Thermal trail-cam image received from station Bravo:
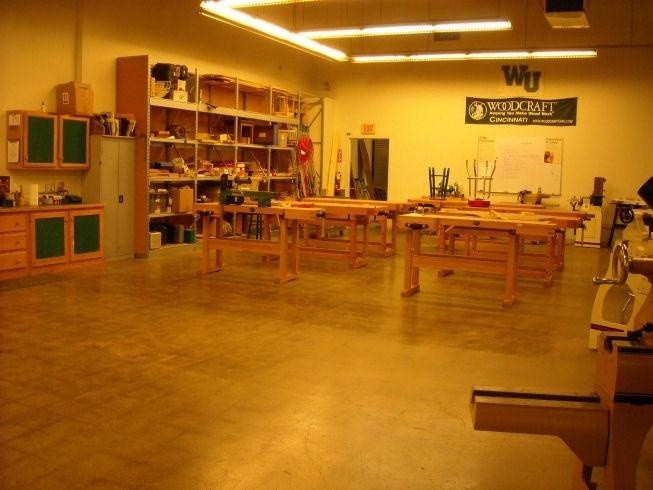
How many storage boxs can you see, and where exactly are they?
[172,187,194,214]
[169,89,188,103]
[149,232,162,250]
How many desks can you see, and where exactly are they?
[184,195,441,282]
[398,198,595,306]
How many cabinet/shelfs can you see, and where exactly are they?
[4,109,91,172]
[113,53,304,261]
[0,209,31,281]
[32,204,106,275]
[81,136,139,264]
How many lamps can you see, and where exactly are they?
[196,0,598,67]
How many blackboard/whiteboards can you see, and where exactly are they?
[475,135,564,196]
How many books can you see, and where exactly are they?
[150,173,178,178]
[150,220,196,245]
[92,109,136,137]
[148,168,169,173]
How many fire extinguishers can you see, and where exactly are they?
[335,172,341,194]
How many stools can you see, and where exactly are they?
[428,164,449,201]
[464,158,497,209]
